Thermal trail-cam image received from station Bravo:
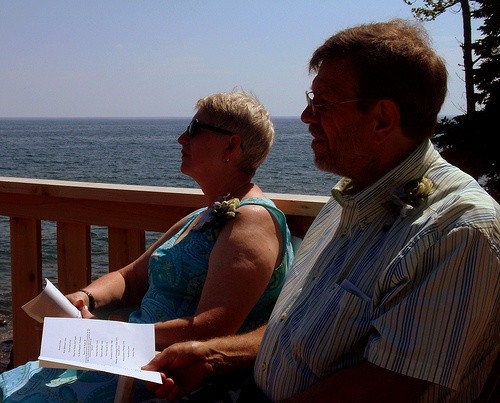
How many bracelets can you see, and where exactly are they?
[79,289,95,313]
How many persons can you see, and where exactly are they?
[0,87,295,403]
[140,19,500,403]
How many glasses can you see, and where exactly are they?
[187,118,245,152]
[305,91,360,116]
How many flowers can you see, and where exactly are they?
[377,176,434,234]
[198,194,241,234]
